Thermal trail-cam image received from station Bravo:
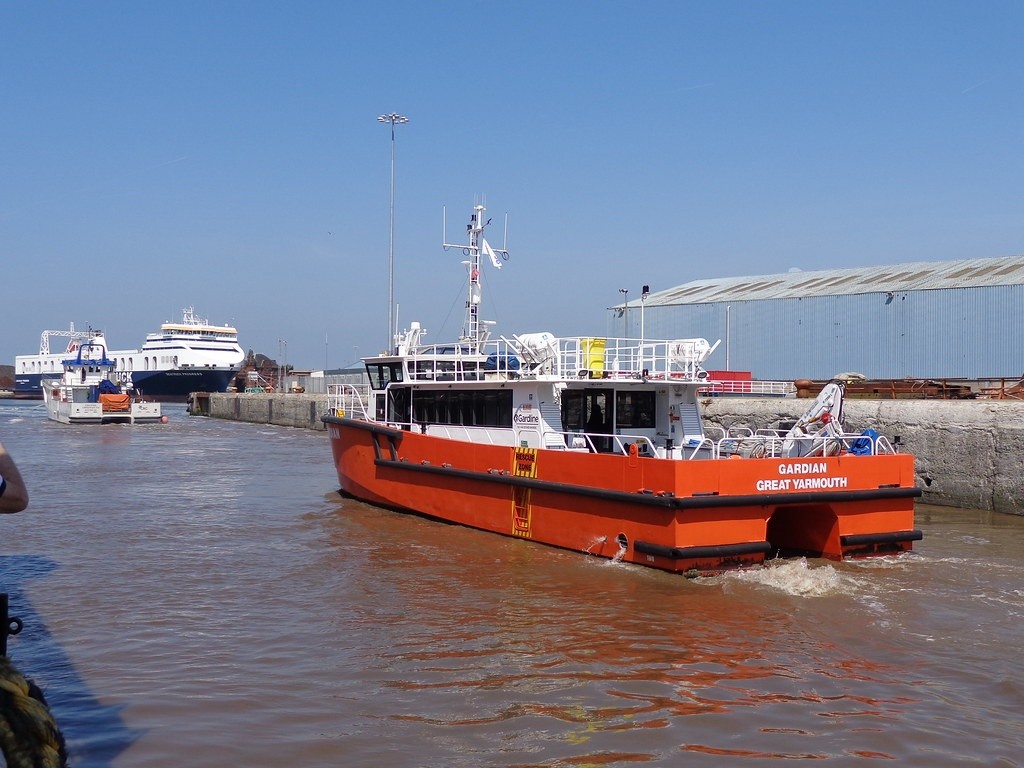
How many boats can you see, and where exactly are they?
[14,305,247,402]
[40,342,162,424]
[321,195,933,576]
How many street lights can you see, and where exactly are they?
[377,112,410,354]
[619,288,628,375]
[277,339,287,393]
